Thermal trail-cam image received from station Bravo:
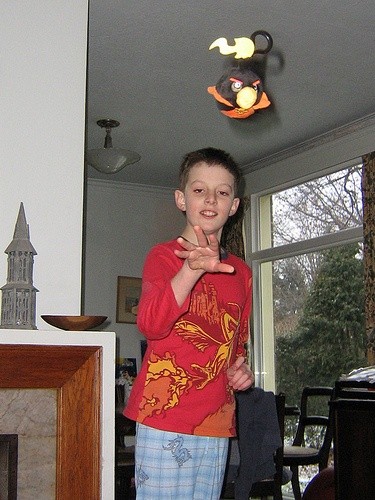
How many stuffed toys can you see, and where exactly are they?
[208,57,271,119]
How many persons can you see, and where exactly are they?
[121,146,255,500]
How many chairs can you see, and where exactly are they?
[253,394,288,500]
[275,385,336,500]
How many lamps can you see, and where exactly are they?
[206,30,274,119]
[85,118,141,175]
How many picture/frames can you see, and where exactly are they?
[115,356,137,407]
[116,275,143,325]
